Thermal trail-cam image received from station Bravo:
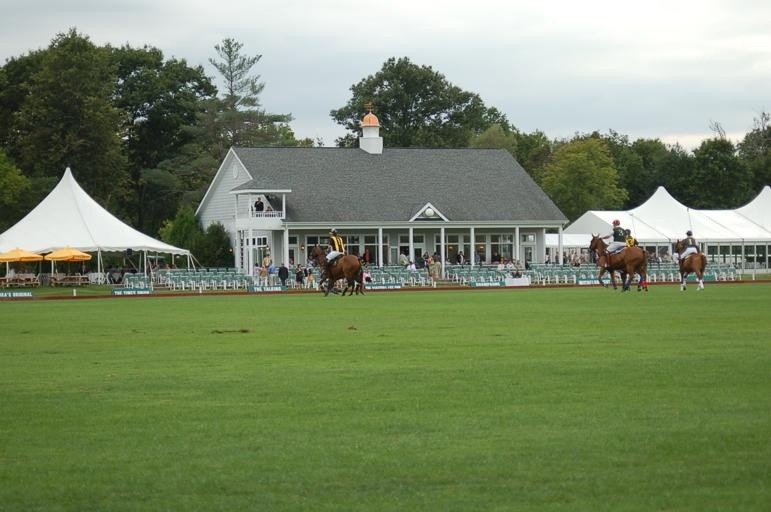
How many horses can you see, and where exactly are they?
[588,233,649,291]
[309,243,366,296]
[674,239,707,292]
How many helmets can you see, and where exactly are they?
[329,229,337,234]
[613,220,619,225]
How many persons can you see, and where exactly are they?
[323,227,344,265]
[396,249,530,277]
[253,197,263,217]
[262,206,275,217]
[363,248,371,266]
[623,229,640,247]
[248,205,253,216]
[102,261,176,281]
[600,219,628,266]
[545,249,675,268]
[251,250,317,288]
[678,230,698,260]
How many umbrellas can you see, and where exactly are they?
[0,248,43,282]
[43,246,92,276]
